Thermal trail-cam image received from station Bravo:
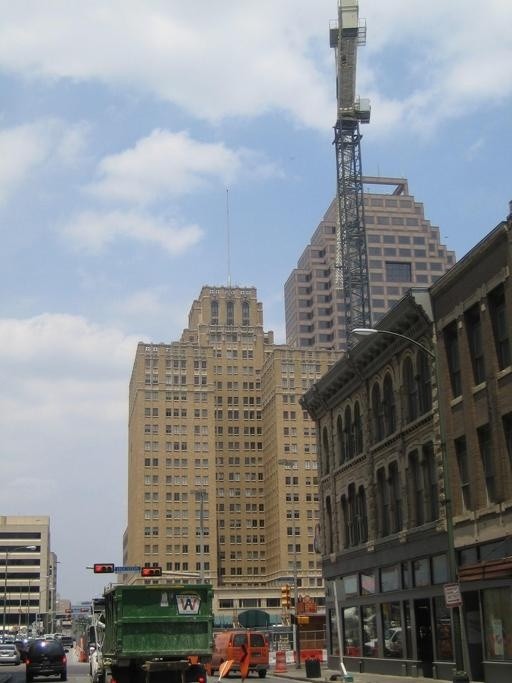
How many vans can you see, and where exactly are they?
[209,629,270,677]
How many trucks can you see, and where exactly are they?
[88,581,216,682]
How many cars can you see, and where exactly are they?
[0,627,101,681]
[365,623,412,658]
[437,621,482,660]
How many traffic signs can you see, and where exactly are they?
[114,567,140,574]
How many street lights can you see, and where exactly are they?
[351,325,434,359]
[2,543,37,644]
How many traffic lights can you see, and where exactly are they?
[140,566,162,576]
[280,583,291,608]
[93,563,115,573]
